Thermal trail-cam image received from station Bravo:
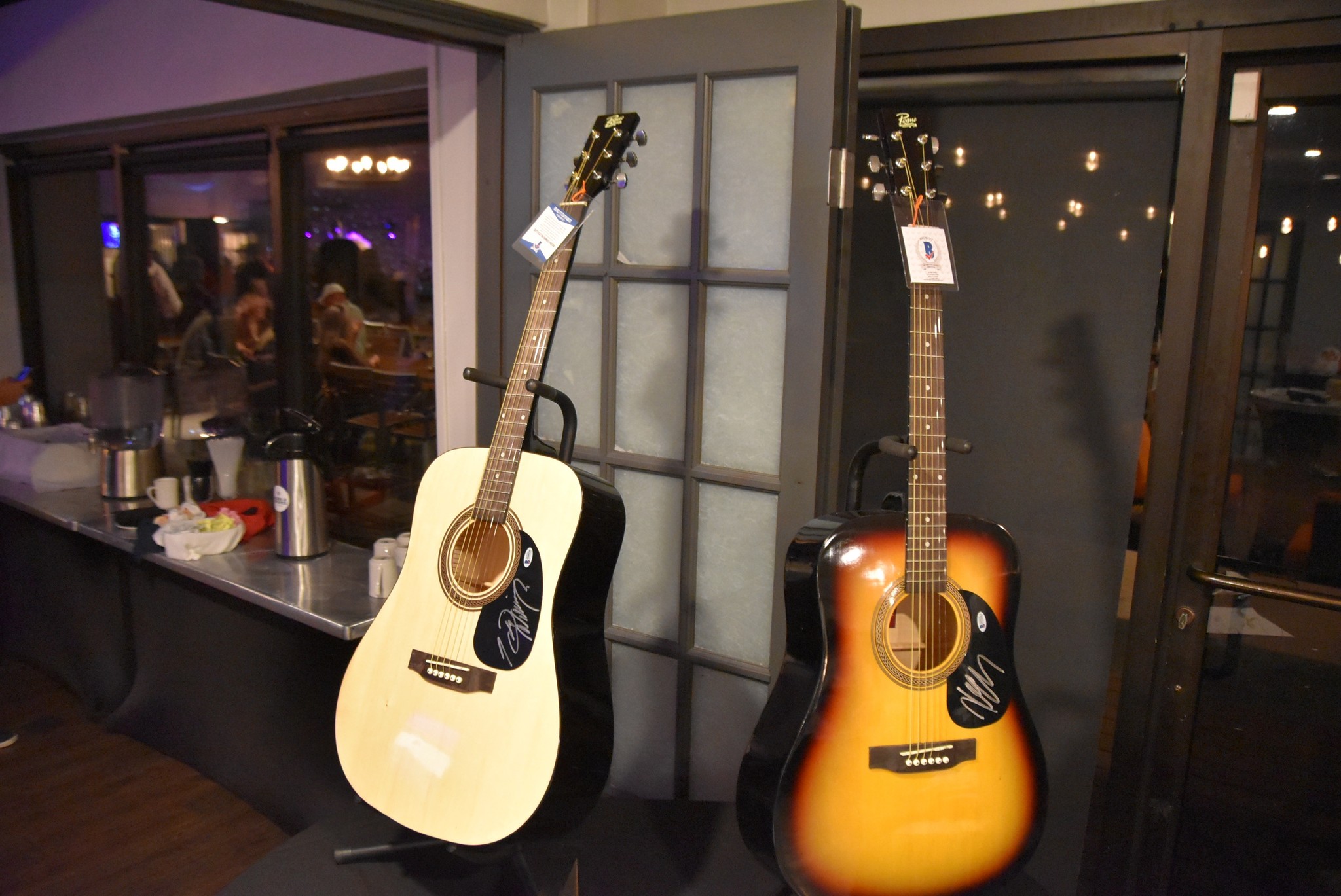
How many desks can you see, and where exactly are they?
[0,485,392,835]
[366,353,436,391]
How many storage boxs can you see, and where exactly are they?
[89,378,159,426]
[0,422,105,491]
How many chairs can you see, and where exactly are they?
[248,321,436,470]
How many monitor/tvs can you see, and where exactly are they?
[100,222,122,249]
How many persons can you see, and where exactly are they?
[113,217,382,368]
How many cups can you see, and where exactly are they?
[205,434,245,503]
[181,475,214,504]
[367,533,410,600]
[146,477,179,510]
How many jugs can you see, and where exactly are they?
[264,432,329,561]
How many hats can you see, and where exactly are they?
[317,283,345,303]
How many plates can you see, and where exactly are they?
[115,522,137,531]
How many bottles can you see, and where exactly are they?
[0,395,47,429]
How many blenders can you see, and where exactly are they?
[86,362,165,499]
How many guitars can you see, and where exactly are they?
[733,103,1052,896]
[331,113,648,847]
